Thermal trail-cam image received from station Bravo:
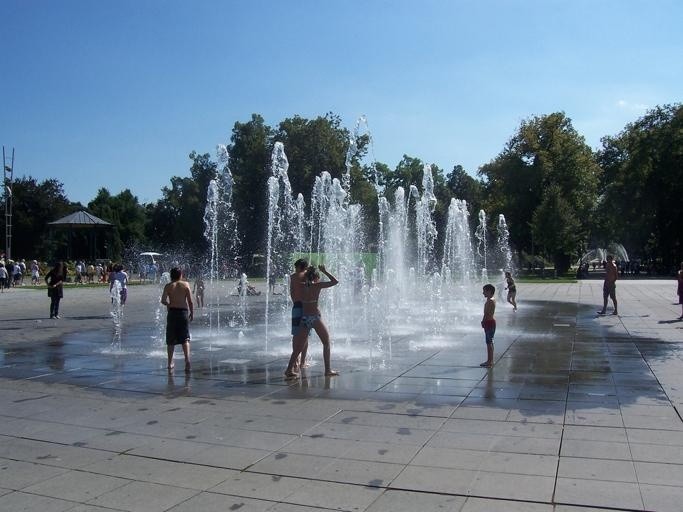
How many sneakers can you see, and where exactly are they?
[50,316,57,319]
[55,315,60,319]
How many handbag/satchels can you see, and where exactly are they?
[15,268,21,279]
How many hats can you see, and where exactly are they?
[10,260,14,263]
[15,262,18,265]
[21,259,25,262]
[34,260,37,263]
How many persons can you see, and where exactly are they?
[128,260,241,284]
[160,267,195,369]
[290,259,309,373]
[597,254,618,315]
[44,262,63,319]
[114,265,128,305]
[576,259,601,280]
[677,262,683,304]
[1,258,27,289]
[504,271,517,311]
[63,260,112,284]
[107,264,119,304]
[480,284,497,367]
[619,260,641,277]
[192,274,205,308]
[30,260,40,284]
[285,263,339,377]
[269,270,276,293]
[237,271,261,296]
